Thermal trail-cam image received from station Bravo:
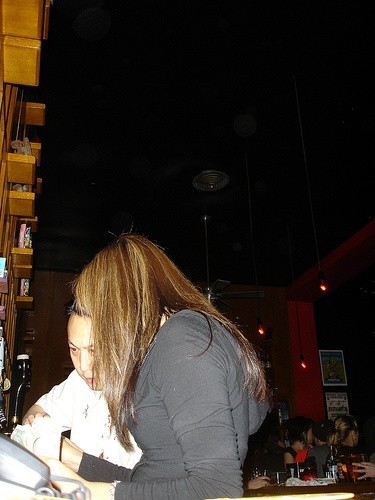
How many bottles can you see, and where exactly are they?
[8,354,32,432]
[326,445,339,484]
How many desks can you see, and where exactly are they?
[244,483,375,500]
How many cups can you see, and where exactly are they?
[276,472,287,484]
[262,470,270,477]
[345,451,370,483]
[303,464,317,481]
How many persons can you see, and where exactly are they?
[23,309,143,471]
[10,237,272,500]
[244,414,375,500]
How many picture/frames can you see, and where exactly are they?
[323,388,351,422]
[318,350,347,386]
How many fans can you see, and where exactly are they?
[193,217,266,311]
